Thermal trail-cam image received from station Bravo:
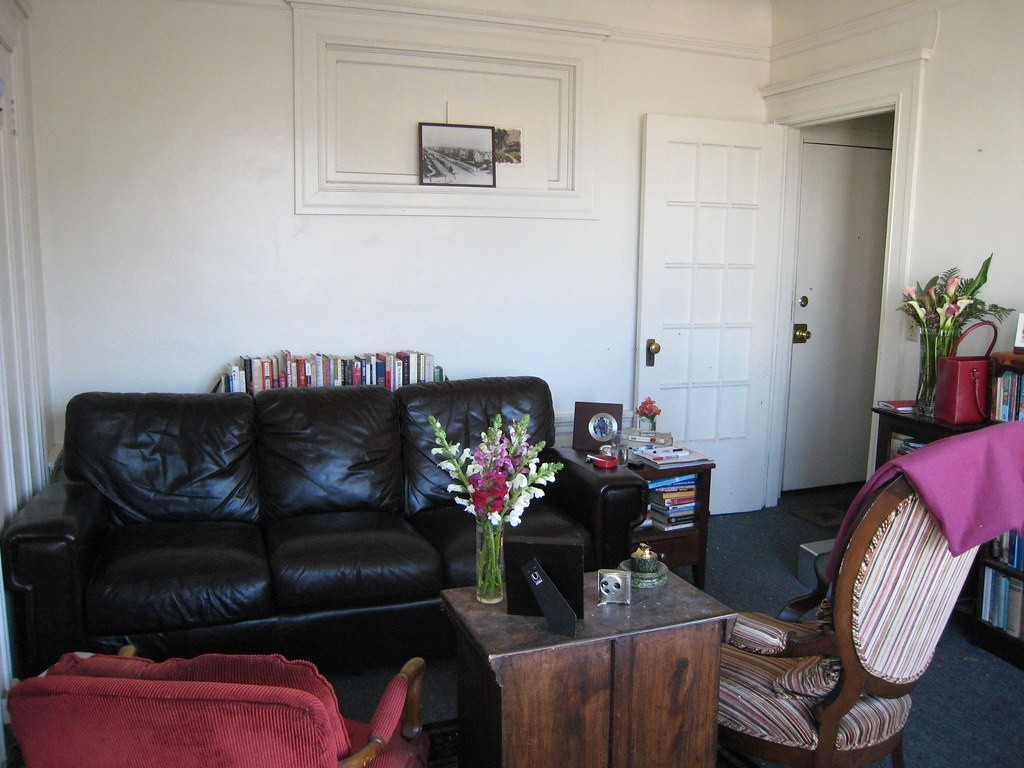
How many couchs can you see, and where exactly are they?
[3,377,591,673]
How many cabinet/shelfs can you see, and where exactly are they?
[868,351,1023,671]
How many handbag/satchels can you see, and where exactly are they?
[934,322,998,426]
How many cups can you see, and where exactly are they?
[611,431,629,466]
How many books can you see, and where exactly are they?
[625,427,715,533]
[219,350,446,391]
[995,372,1024,423]
[982,529,1023,638]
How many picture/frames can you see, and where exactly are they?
[504,536,584,619]
[419,122,496,188]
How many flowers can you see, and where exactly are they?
[895,252,1017,410]
[428,413,565,598]
[636,397,662,431]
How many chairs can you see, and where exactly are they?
[7,647,431,768]
[713,422,1024,768]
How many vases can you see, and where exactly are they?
[475,518,504,603]
[648,420,657,431]
[914,328,960,417]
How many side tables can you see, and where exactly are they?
[548,438,715,589]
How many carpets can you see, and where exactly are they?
[792,500,852,535]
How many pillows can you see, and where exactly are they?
[45,648,356,760]
[772,599,865,702]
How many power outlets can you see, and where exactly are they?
[905,317,921,341]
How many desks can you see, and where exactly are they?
[441,558,736,768]
